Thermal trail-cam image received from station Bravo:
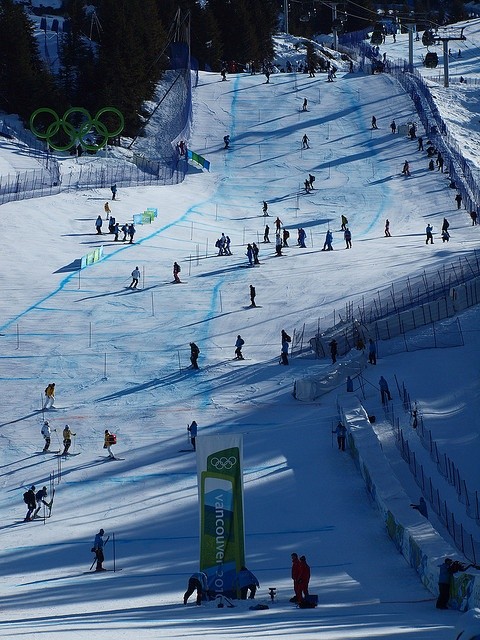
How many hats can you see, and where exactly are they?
[100,529,104,533]
[300,556,306,560]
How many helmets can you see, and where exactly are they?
[44,421,49,424]
[65,424,69,429]
[43,486,47,490]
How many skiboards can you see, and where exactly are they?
[226,357,252,361]
[99,456,125,461]
[124,242,139,244]
[33,407,65,412]
[239,265,260,268]
[167,282,188,284]
[269,255,287,257]
[54,453,80,456]
[32,516,50,518]
[35,450,60,453]
[179,449,195,451]
[84,568,122,574]
[15,519,46,522]
[243,305,262,309]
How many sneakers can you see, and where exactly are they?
[50,406,55,408]
[43,407,48,409]
[96,567,103,571]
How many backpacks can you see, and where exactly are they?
[299,599,317,608]
[109,434,117,444]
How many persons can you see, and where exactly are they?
[435,558,454,610]
[187,420,198,449]
[189,342,200,369]
[332,420,347,451]
[178,140,186,155]
[234,335,244,359]
[273,217,283,233]
[109,216,115,234]
[427,140,434,145]
[449,179,458,189]
[328,337,338,363]
[173,262,182,283]
[371,116,378,129]
[279,337,289,365]
[223,135,232,149]
[390,121,396,133]
[282,228,289,247]
[237,565,260,600]
[281,329,287,345]
[300,230,306,248]
[104,202,111,220]
[297,228,301,245]
[410,126,416,141]
[436,157,445,173]
[111,184,117,200]
[23,484,36,522]
[40,420,57,454]
[426,146,435,156]
[128,265,141,289]
[460,76,464,83]
[442,217,449,235]
[290,553,303,605]
[225,236,231,254]
[323,230,331,250]
[103,429,117,461]
[455,195,462,209]
[93,529,106,571]
[250,284,257,307]
[434,147,440,153]
[276,233,282,256]
[418,137,424,151]
[379,375,392,403]
[302,133,310,149]
[441,231,450,243]
[401,159,410,176]
[356,338,365,350]
[449,48,452,56]
[385,219,391,237]
[183,572,209,605]
[61,424,76,456]
[471,210,478,226]
[264,224,270,242]
[308,173,315,190]
[218,232,229,255]
[457,48,462,56]
[346,375,353,393]
[112,223,121,241]
[33,486,53,519]
[122,224,128,240]
[392,33,396,44]
[436,150,441,166]
[252,242,259,264]
[128,224,135,243]
[344,228,352,249]
[296,555,310,597]
[246,243,254,266]
[95,215,102,234]
[368,338,376,365]
[303,178,310,194]
[302,97,308,111]
[217,44,387,84]
[426,224,434,244]
[428,158,435,171]
[340,214,347,230]
[262,200,269,216]
[43,382,56,410]
